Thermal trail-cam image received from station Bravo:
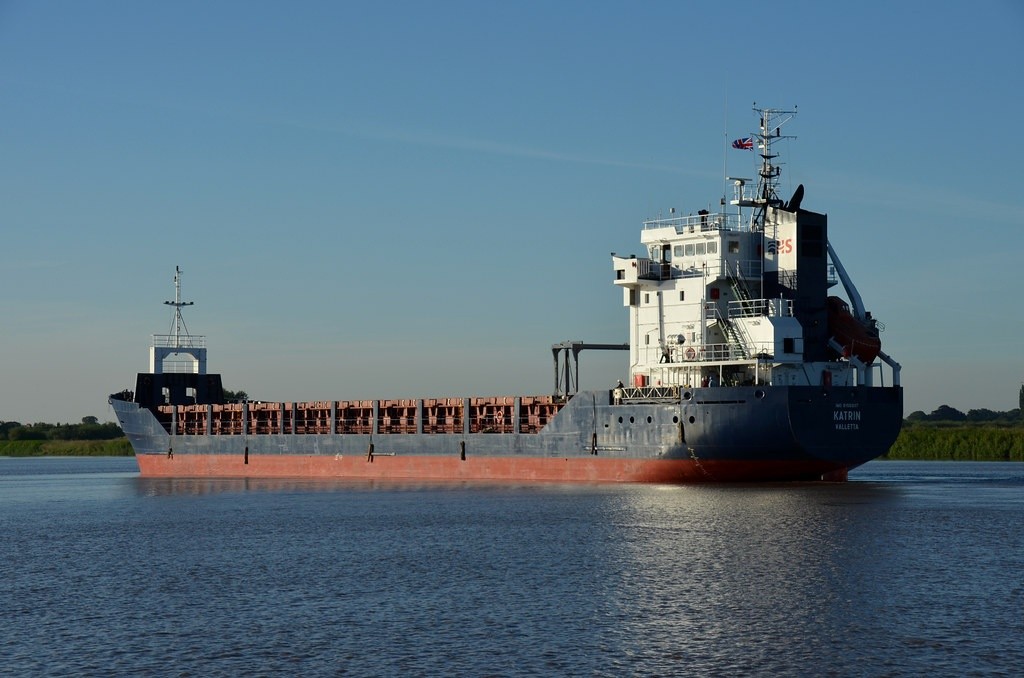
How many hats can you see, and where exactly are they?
[617,380,621,383]
[709,376,712,380]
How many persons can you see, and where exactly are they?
[616,380,624,388]
[702,376,719,388]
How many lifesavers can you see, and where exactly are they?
[685,348,695,359]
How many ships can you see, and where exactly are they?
[108,91,904,481]
